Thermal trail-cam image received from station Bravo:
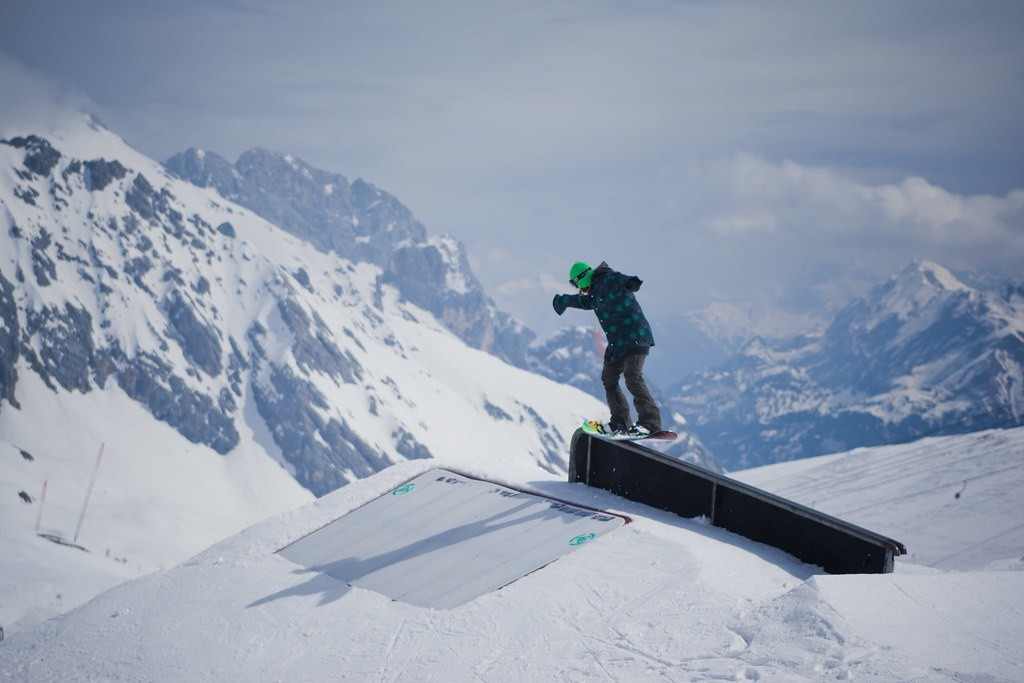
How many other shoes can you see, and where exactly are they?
[620,421,651,437]
[595,422,625,436]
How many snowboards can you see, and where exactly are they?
[581,419,678,441]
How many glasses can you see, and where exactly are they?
[569,279,578,288]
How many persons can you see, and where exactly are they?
[553,261,661,435]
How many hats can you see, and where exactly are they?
[569,261,594,289]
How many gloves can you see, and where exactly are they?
[626,276,643,292]
